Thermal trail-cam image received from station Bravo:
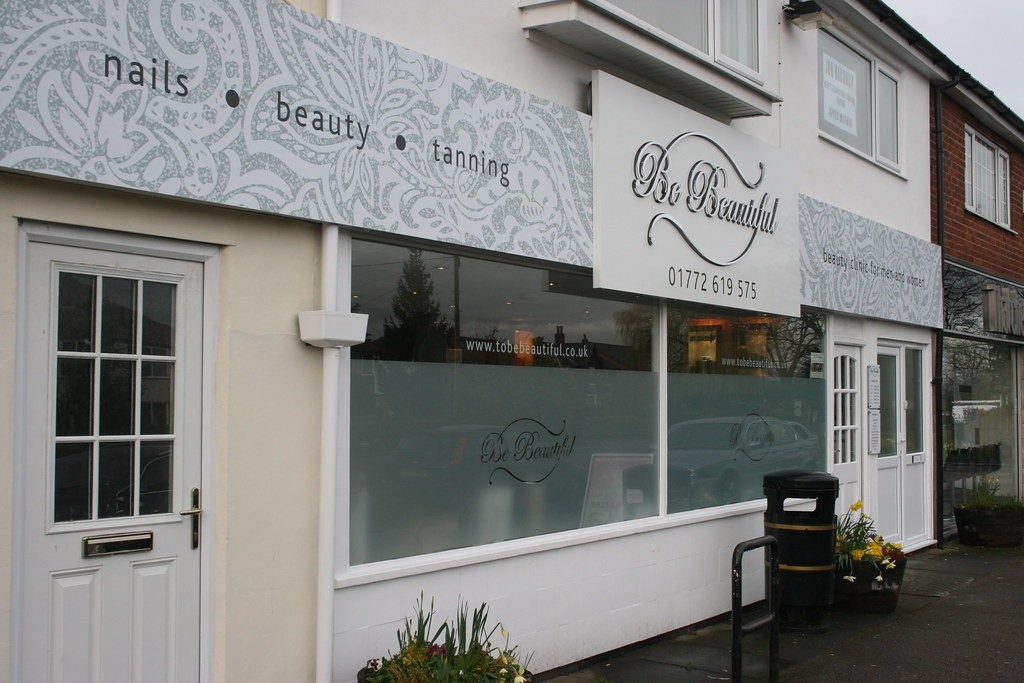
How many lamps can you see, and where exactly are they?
[515,328,535,367]
[783,0,834,31]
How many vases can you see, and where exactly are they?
[953,505,1024,548]
[834,558,907,615]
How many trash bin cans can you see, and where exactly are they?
[761,471,839,631]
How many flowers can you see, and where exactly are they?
[973,472,1001,507]
[835,500,908,583]
[356,590,540,683]
